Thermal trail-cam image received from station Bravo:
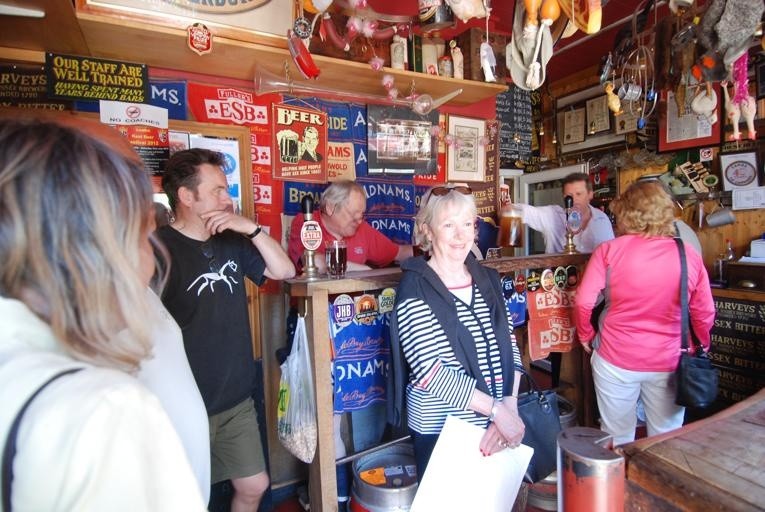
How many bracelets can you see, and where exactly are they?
[246,223,262,238]
[509,394,519,399]
[487,398,503,423]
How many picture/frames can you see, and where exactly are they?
[445,112,487,184]
[717,149,762,192]
[656,78,725,156]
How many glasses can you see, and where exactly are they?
[343,205,364,222]
[425,186,472,207]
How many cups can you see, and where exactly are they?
[495,204,524,249]
[705,202,735,228]
[325,240,347,279]
[279,136,301,164]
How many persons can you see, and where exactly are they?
[145,148,298,512]
[285,177,420,512]
[625,177,704,426]
[495,169,617,392]
[298,125,324,163]
[572,177,717,449]
[51,115,216,512]
[394,181,534,512]
[0,109,213,512]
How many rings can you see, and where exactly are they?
[503,441,508,449]
[498,438,504,445]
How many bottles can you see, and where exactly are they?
[390,34,405,70]
[694,201,705,230]
[420,31,453,78]
[712,239,737,285]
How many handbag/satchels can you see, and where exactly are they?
[513,363,562,485]
[675,347,719,410]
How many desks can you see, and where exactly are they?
[281,246,593,511]
